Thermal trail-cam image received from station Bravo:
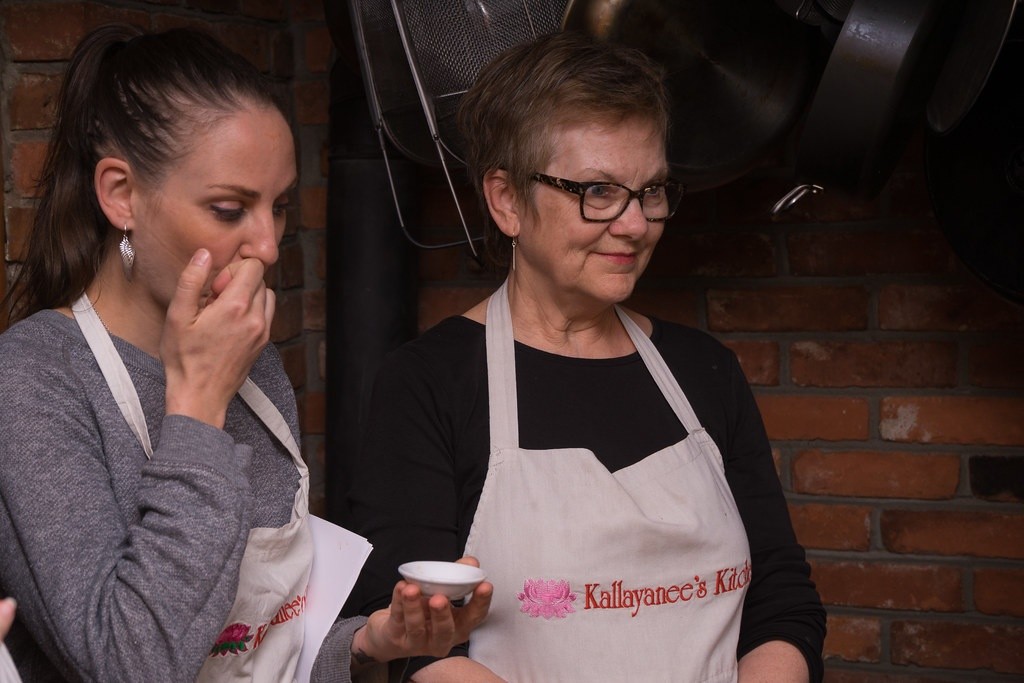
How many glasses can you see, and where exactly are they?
[529,168,685,221]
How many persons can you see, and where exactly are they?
[312,33,827,683]
[0,24,493,683]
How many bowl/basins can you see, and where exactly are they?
[398,560,487,601]
